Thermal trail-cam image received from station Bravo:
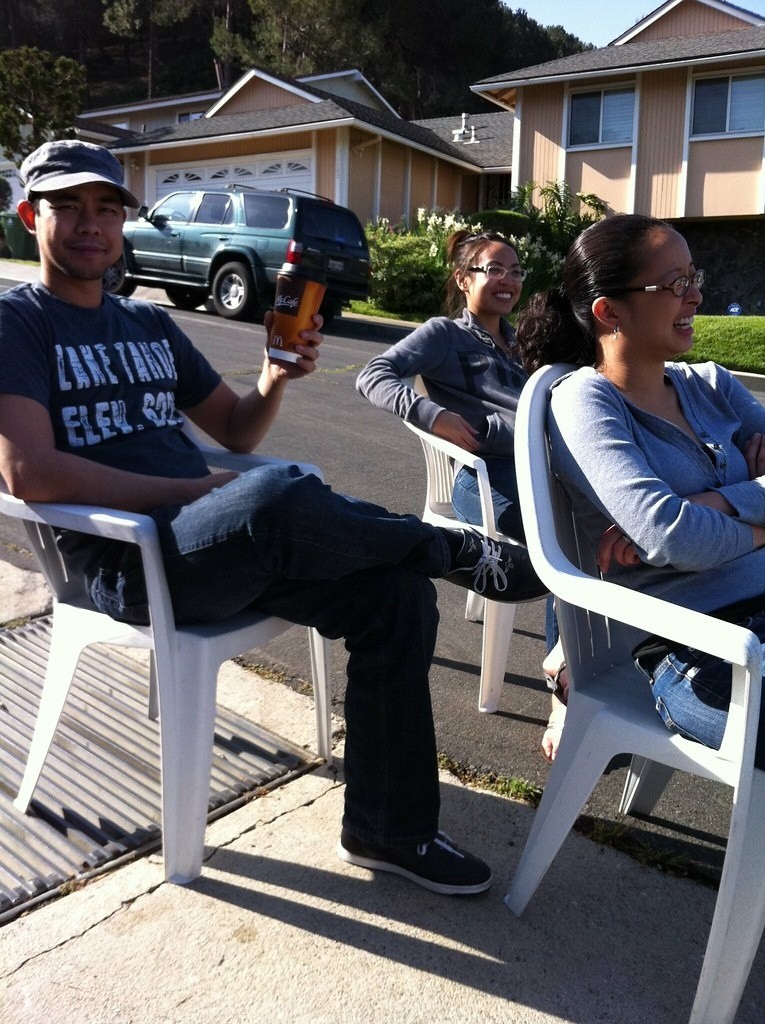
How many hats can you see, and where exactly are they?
[19,139,139,211]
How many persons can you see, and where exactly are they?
[549,213,763,770]
[1,139,553,895]
[356,230,606,765]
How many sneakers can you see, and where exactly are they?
[340,827,493,895]
[441,526,554,605]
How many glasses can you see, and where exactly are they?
[461,266,527,281]
[621,268,705,296]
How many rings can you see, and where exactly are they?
[622,533,632,544]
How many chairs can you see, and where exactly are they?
[211,204,226,221]
[0,406,333,885]
[505,359,765,1023]
[398,367,526,714]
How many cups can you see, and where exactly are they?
[267,259,330,365]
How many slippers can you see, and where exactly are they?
[541,661,569,705]
[539,720,566,764]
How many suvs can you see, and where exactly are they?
[102,185,378,326]
